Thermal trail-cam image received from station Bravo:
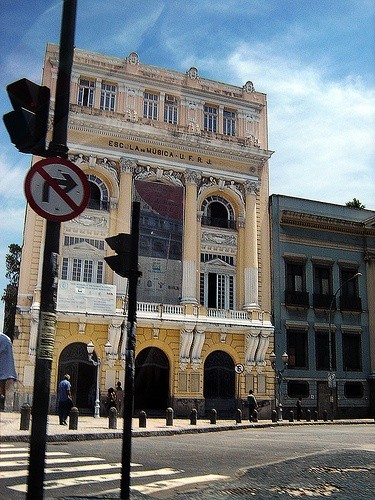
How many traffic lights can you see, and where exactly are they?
[103,233,131,277]
[2,77,51,155]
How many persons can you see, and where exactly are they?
[247,390,258,421]
[58,374,73,426]
[295,400,302,422]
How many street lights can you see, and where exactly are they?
[328,273,362,420]
[86,340,112,418]
[269,351,288,420]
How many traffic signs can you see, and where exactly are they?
[234,363,244,374]
